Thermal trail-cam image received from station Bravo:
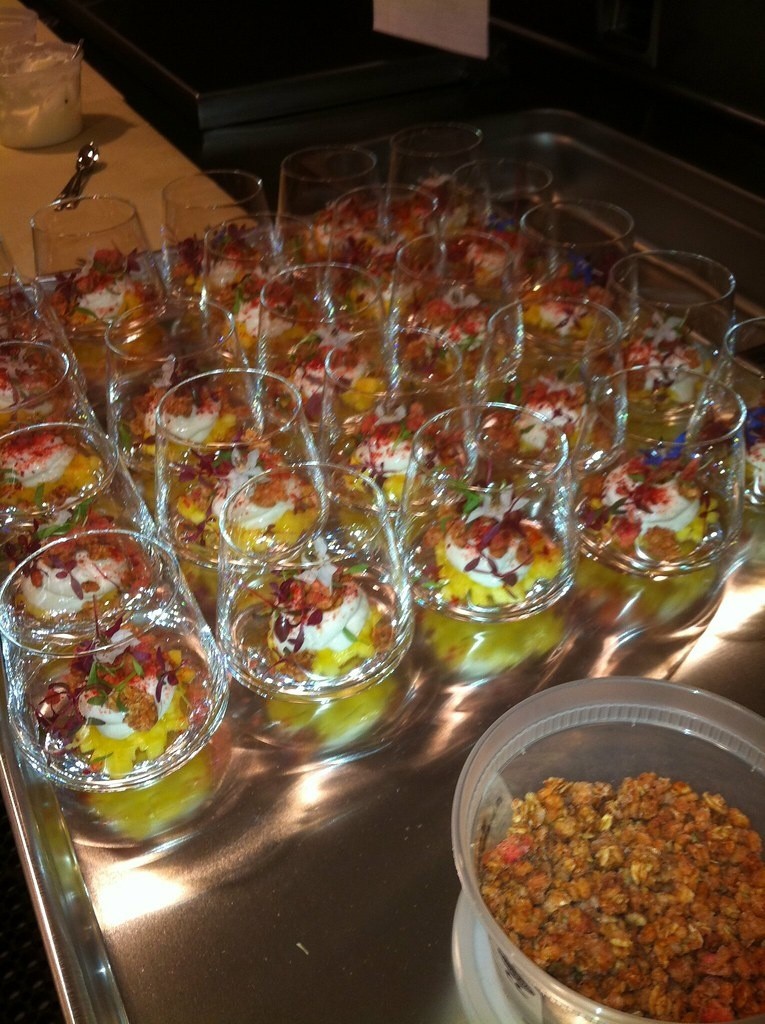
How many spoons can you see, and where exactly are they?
[65,144,99,208]
[50,143,94,211]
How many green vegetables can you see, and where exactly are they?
[0,197,699,763]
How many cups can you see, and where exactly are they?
[0,4,38,54]
[1,117,764,850]
[0,39,82,149]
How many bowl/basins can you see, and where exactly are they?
[452,675,765,1023]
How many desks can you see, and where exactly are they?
[1,0,257,279]
